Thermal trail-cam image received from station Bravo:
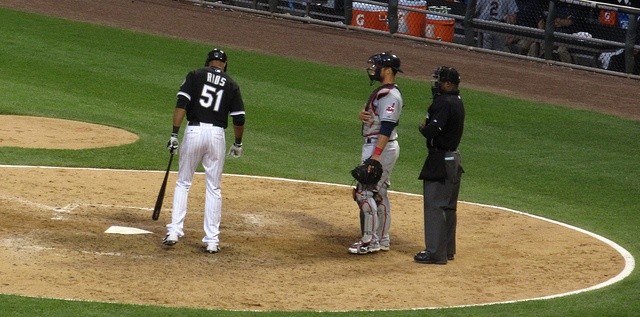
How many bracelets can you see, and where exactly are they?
[173,126,179,133]
[373,146,383,155]
[235,137,241,144]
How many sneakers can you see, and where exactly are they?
[379,238,389,251]
[348,241,380,253]
[205,243,218,253]
[162,234,178,246]
[416,250,453,260]
[414,254,448,264]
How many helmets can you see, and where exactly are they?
[205,48,227,72]
[366,51,400,80]
[430,66,461,95]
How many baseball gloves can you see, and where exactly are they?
[350,159,383,185]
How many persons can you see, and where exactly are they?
[162,48,245,253]
[527,2,576,63]
[348,50,404,255]
[473,0,519,52]
[414,65,465,265]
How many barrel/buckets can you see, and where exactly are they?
[397,0,425,37]
[350,2,387,30]
[424,14,454,42]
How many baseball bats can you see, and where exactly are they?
[153,154,173,220]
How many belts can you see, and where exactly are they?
[188,121,216,126]
[364,133,398,143]
[428,147,458,152]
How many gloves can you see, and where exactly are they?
[166,133,178,156]
[228,142,243,158]
